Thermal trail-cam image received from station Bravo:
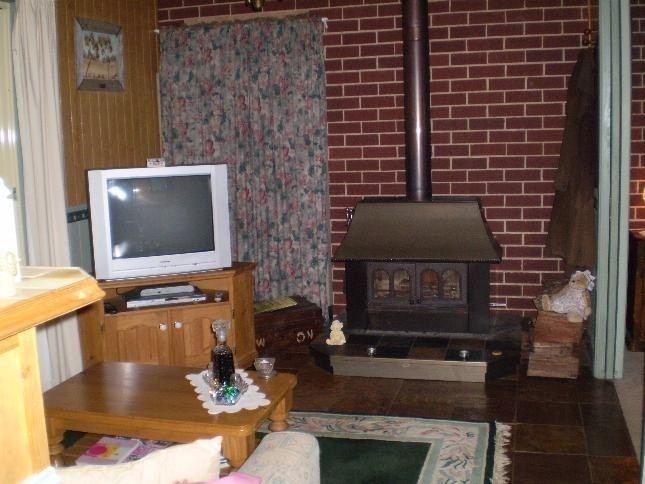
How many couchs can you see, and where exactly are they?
[22,431,321,483]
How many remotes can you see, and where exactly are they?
[104,302,116,313]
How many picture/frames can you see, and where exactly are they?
[73,16,125,92]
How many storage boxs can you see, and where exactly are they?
[521,305,589,380]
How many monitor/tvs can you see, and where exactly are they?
[87,162,233,281]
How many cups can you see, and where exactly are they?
[254,356,275,379]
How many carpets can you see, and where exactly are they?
[62,410,514,484]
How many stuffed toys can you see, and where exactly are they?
[542,269,595,322]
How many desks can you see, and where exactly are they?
[626,229,645,352]
[0,264,106,483]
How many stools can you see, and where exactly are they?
[253,295,325,365]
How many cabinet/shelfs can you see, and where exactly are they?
[78,261,258,375]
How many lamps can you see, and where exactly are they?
[247,0,276,10]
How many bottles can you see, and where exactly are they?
[210,319,235,387]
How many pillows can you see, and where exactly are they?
[61,436,224,483]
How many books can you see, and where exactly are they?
[76,436,164,467]
